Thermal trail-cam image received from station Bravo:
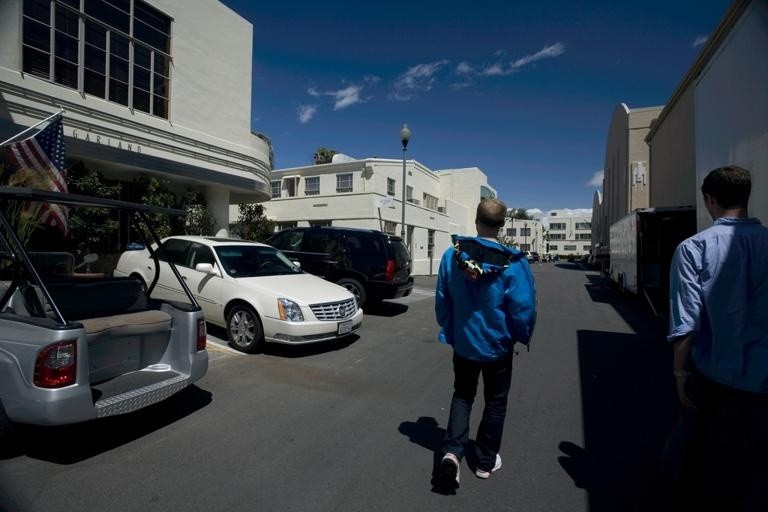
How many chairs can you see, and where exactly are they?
[21,253,141,317]
[240,249,271,272]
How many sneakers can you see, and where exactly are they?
[475,454,502,479]
[440,453,460,489]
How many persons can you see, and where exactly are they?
[434,197,539,491]
[665,164,768,512]
[543,252,560,266]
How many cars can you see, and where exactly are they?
[0,195,210,426]
[113,235,364,354]
[523,251,560,264]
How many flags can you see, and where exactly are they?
[6,115,69,238]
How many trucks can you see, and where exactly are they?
[609,207,696,317]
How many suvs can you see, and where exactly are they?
[255,225,412,307]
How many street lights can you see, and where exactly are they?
[400,123,411,243]
[524,222,527,251]
